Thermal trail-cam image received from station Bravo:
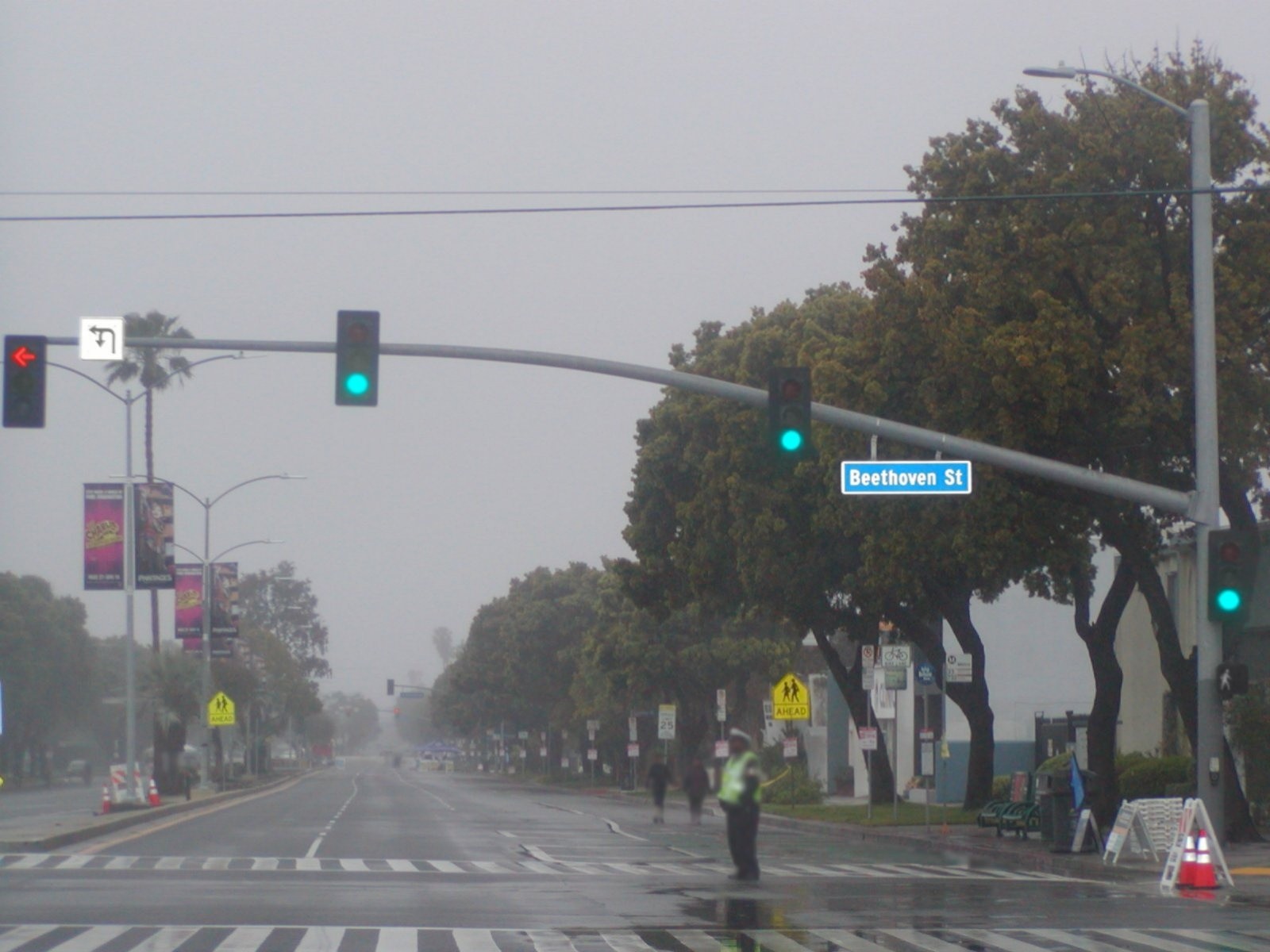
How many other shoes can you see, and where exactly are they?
[654,816,664,824]
[692,819,701,826]
[726,870,761,881]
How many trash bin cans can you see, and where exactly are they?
[1033,765,1099,854]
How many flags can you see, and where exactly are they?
[81,482,126,591]
[173,563,203,638]
[182,636,205,657]
[134,480,178,591]
[210,636,235,657]
[208,560,241,640]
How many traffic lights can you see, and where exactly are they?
[1208,529,1258,623]
[2,334,47,430]
[387,679,394,696]
[767,365,813,460]
[335,309,381,407]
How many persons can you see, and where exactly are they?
[646,753,679,826]
[717,726,763,880]
[682,756,712,827]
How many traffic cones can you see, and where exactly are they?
[1175,831,1197,884]
[1194,829,1222,889]
[147,778,164,807]
[100,785,113,815]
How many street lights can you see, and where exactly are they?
[1023,64,1228,825]
[51,350,317,800]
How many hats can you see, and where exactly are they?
[730,728,750,743]
